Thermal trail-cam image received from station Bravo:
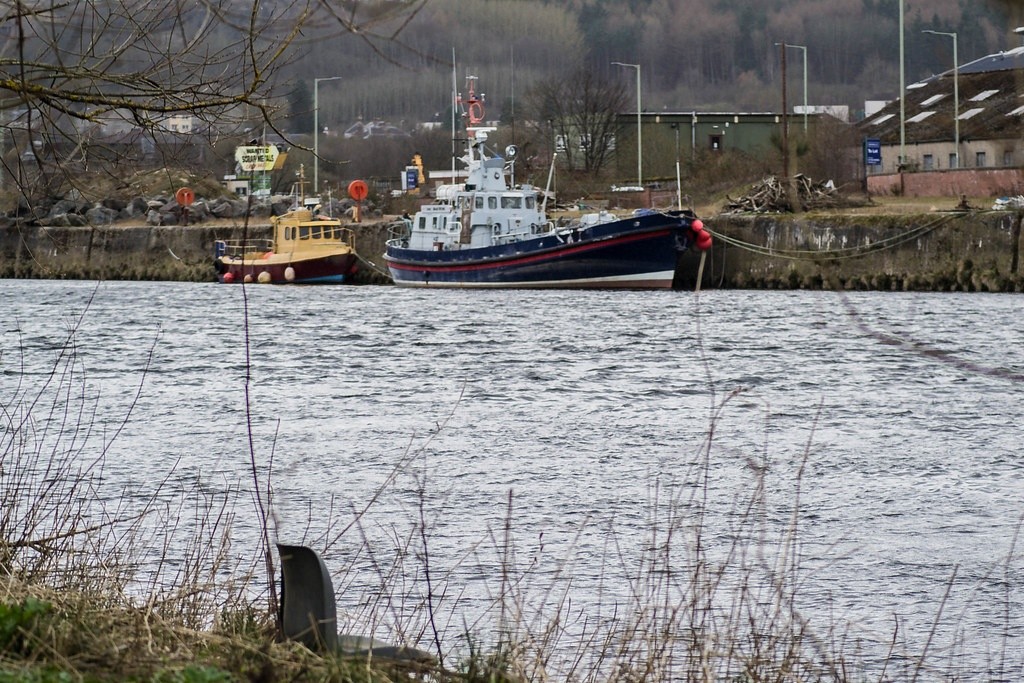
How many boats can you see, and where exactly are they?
[383,46,712,294]
[215,165,359,285]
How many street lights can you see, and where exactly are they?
[774,42,808,136]
[922,29,959,168]
[609,60,642,191]
[314,76,344,199]
